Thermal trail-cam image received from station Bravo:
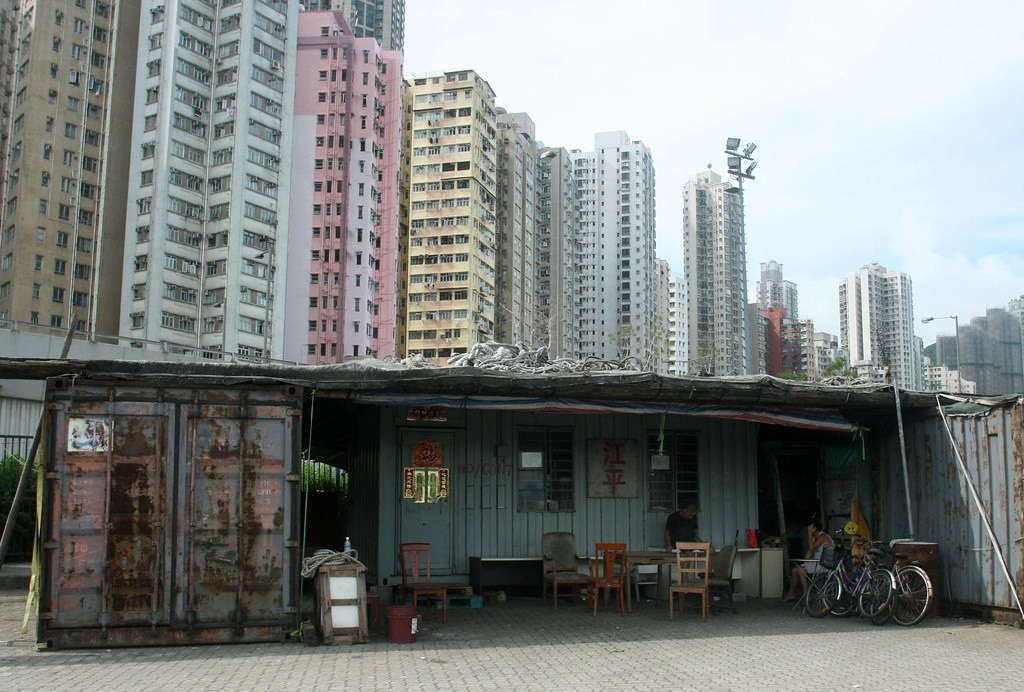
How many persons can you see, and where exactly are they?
[782,520,835,602]
[665,501,714,583]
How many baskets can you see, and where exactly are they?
[819,544,845,570]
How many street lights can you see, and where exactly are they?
[724,137,758,375]
[922,315,962,393]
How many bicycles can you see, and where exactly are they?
[804,526,933,626]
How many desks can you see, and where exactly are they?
[367,593,381,630]
[785,559,840,614]
[390,582,473,611]
[575,547,762,606]
[468,556,542,600]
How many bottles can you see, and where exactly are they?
[344,537,351,556]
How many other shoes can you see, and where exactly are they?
[780,595,797,602]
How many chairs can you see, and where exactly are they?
[669,542,739,620]
[586,543,629,618]
[543,532,594,611]
[397,543,446,624]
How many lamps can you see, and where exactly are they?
[403,406,447,422]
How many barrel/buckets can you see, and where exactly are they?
[894,541,939,620]
[386,606,418,643]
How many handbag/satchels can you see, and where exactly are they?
[746,528,758,547]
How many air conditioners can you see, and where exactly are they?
[25,10,32,16]
[44,173,50,177]
[56,11,63,17]
[18,66,23,72]
[45,145,52,150]
[10,172,17,177]
[89,2,283,318]
[14,144,19,150]
[52,63,59,71]
[47,117,54,123]
[51,90,58,95]
[54,37,61,45]
